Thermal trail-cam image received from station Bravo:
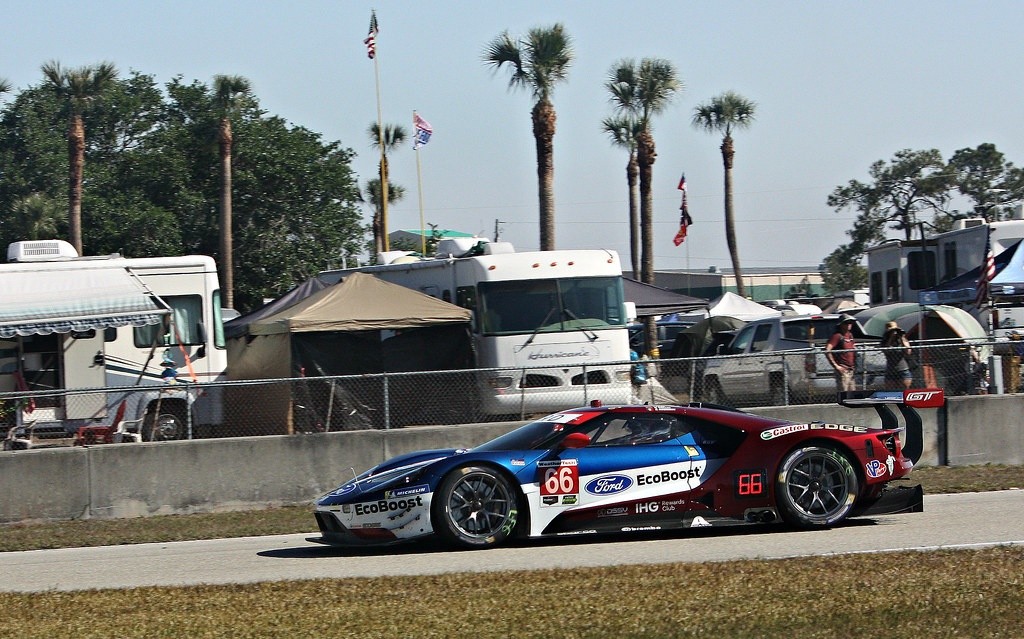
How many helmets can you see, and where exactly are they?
[621,416,672,446]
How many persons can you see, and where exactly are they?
[826,313,858,391]
[880,321,913,389]
[622,416,677,444]
[1005,331,1024,393]
[957,341,989,396]
[290,341,328,433]
[630,345,642,402]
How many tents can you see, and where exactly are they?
[223,274,483,437]
[621,275,782,393]
[918,238,1024,364]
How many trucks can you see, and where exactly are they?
[866,218,1023,355]
[0,239,228,443]
[316,235,637,418]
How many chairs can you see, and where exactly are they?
[112,394,149,443]
[78,400,127,444]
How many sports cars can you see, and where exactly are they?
[313,386,948,554]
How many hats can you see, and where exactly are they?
[836,314,857,326]
[885,322,902,332]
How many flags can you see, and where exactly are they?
[673,174,693,246]
[363,8,380,59]
[974,224,996,308]
[413,112,433,150]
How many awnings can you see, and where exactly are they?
[0,270,173,385]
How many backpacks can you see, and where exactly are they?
[631,365,647,385]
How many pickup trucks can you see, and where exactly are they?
[702,312,889,409]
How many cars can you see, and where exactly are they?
[626,319,698,359]
